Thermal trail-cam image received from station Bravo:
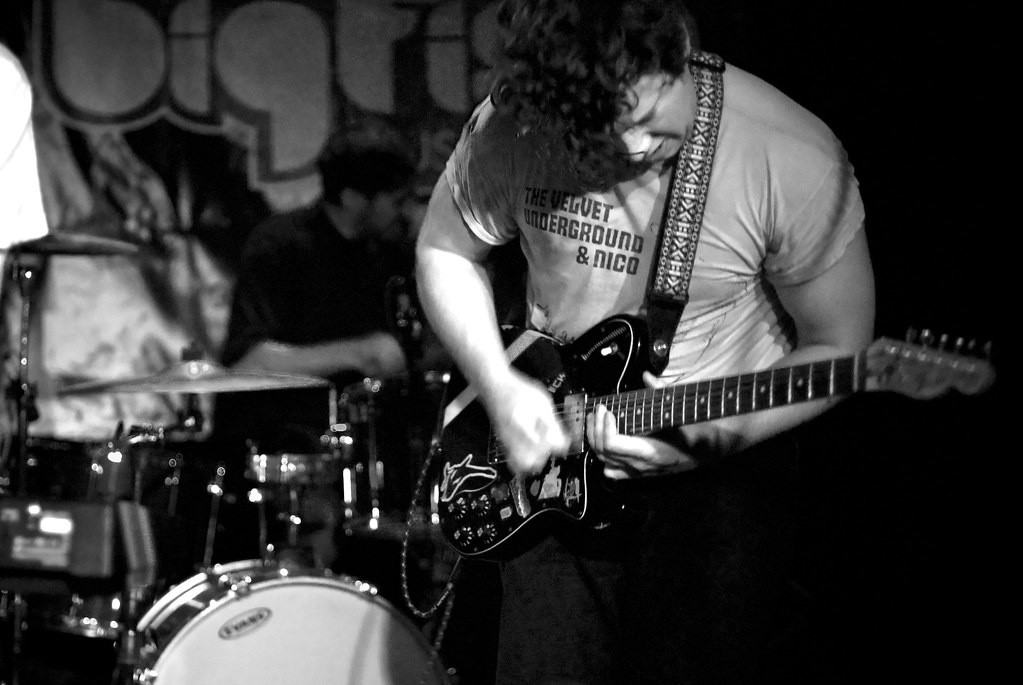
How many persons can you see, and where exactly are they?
[413,0,876,685]
[211,140,438,566]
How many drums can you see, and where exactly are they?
[132,424,361,571]
[1,434,186,637]
[109,556,458,685]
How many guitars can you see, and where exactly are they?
[437,311,995,561]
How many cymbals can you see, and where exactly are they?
[0,228,138,255]
[55,358,329,394]
